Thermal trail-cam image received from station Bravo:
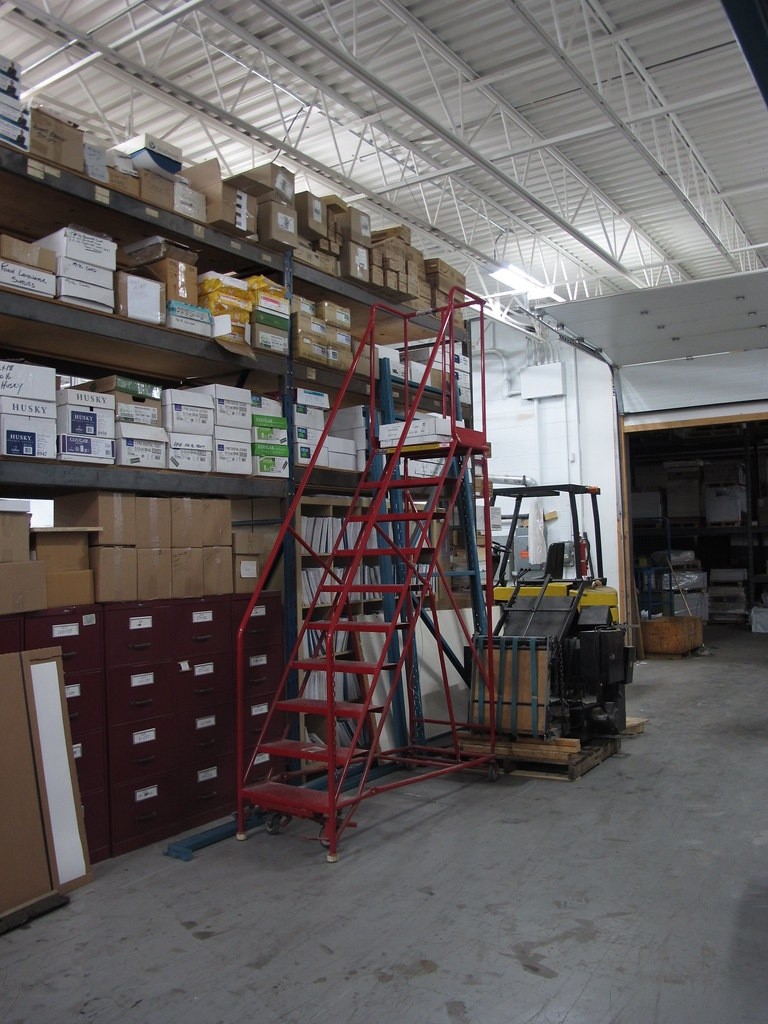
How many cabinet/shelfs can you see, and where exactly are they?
[631,443,768,624]
[0,590,285,863]
[296,497,452,779]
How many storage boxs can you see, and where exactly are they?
[0,226,453,391]
[641,616,705,653]
[0,491,281,613]
[0,55,465,330]
[1,361,461,480]
